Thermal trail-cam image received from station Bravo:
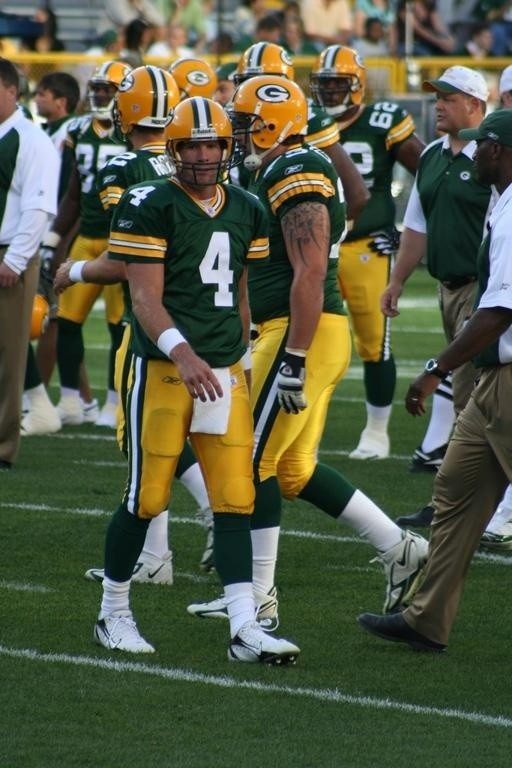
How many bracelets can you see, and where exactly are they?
[238,347,252,373]
[155,326,187,359]
[68,260,86,284]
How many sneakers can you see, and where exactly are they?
[20,399,119,435]
[350,428,390,460]
[409,444,449,473]
[357,505,447,651]
[84,508,301,665]
[478,531,512,551]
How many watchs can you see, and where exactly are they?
[424,358,448,381]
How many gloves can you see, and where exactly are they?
[367,227,401,256]
[278,347,306,414]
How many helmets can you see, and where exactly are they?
[88,42,366,185]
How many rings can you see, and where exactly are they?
[411,397,419,402]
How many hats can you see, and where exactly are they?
[424,66,512,154]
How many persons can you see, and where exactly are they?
[0,0,510,549]
[94,96,302,668]
[51,62,222,584]
[189,74,434,632]
[353,108,512,655]
